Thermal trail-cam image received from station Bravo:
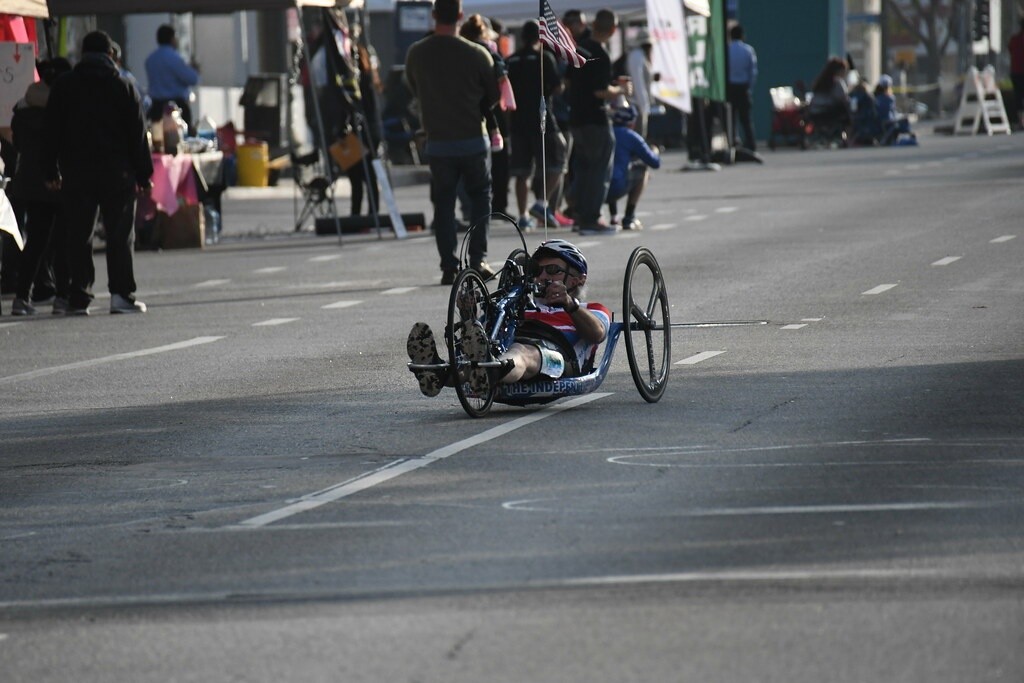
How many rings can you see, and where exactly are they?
[554,292,559,297]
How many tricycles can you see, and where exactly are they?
[405,211,672,419]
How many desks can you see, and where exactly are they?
[136,152,226,247]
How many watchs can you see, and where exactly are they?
[563,296,579,314]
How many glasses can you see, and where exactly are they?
[533,264,575,278]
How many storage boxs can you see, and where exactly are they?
[218,183,313,232]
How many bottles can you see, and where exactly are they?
[203,205,218,244]
[198,113,218,152]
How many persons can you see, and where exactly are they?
[0,8,924,317]
[1009,16,1024,131]
[405,0,501,282]
[407,239,611,397]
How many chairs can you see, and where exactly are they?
[287,146,335,231]
[770,85,827,148]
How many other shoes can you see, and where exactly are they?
[529,203,559,228]
[461,319,490,394]
[429,216,470,234]
[518,217,536,232]
[70,307,90,316]
[110,294,147,314]
[491,134,504,151]
[30,293,56,306]
[609,216,621,225]
[571,217,620,236]
[11,299,35,316]
[621,219,643,230]
[406,322,448,398]
[469,255,498,281]
[441,267,459,285]
[537,213,574,228]
[52,298,68,313]
[490,211,517,221]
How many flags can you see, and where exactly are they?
[684,0,725,103]
[538,0,600,68]
[645,0,691,116]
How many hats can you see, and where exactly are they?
[613,106,639,124]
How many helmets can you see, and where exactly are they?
[531,239,587,273]
